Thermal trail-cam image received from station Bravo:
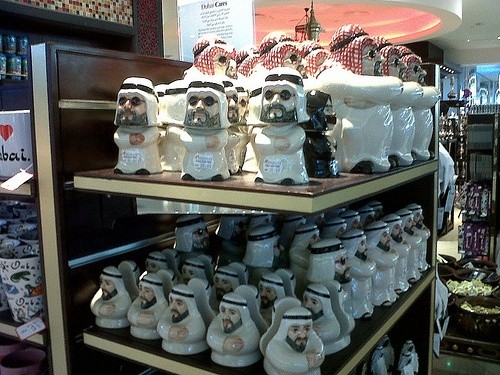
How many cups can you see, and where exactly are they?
[0,193,46,324]
[0,109,34,181]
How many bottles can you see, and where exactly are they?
[447,75,457,98]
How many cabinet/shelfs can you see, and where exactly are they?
[1,0,499,375]
